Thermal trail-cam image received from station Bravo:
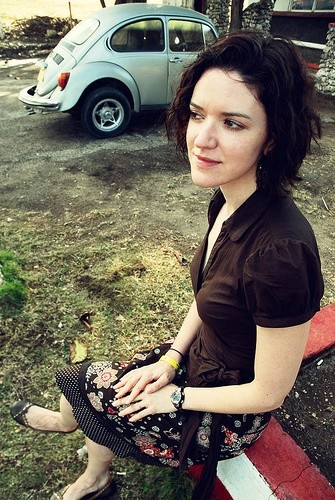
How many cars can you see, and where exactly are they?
[16,3,220,139]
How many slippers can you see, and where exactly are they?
[50,477,115,500]
[10,401,78,433]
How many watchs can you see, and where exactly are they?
[169,386,186,412]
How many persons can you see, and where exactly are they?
[11,30,325,500]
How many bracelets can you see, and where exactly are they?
[169,348,185,359]
[160,355,180,371]
